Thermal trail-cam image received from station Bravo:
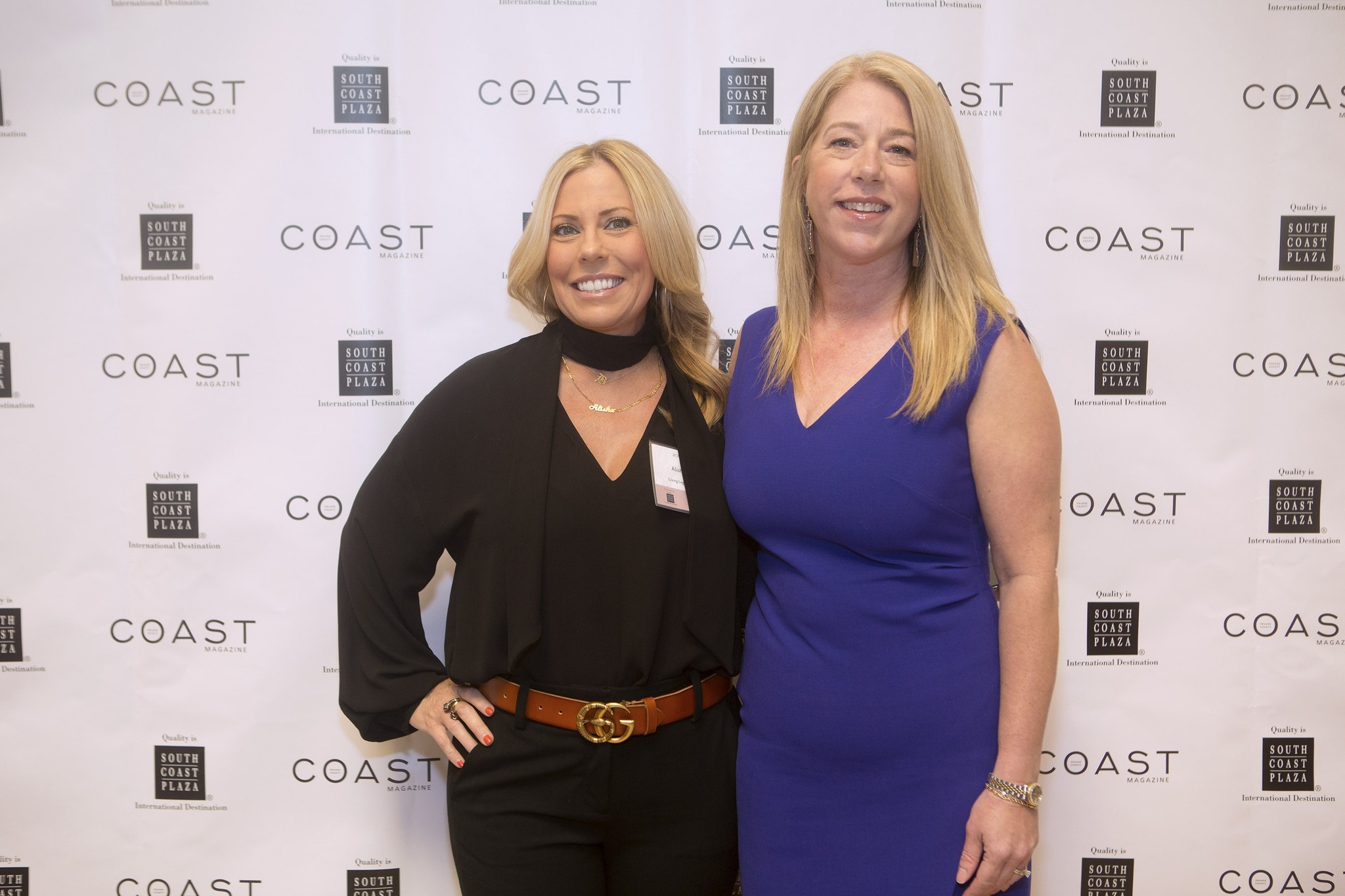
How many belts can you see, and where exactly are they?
[479,673,733,743]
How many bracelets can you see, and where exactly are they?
[984,782,1037,810]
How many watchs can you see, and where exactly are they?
[986,772,1043,806]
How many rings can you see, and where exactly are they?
[1013,865,1031,878]
[443,698,465,720]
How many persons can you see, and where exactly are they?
[721,54,1063,896]
[337,141,759,896]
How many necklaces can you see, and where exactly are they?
[561,344,662,413]
[582,359,644,385]
